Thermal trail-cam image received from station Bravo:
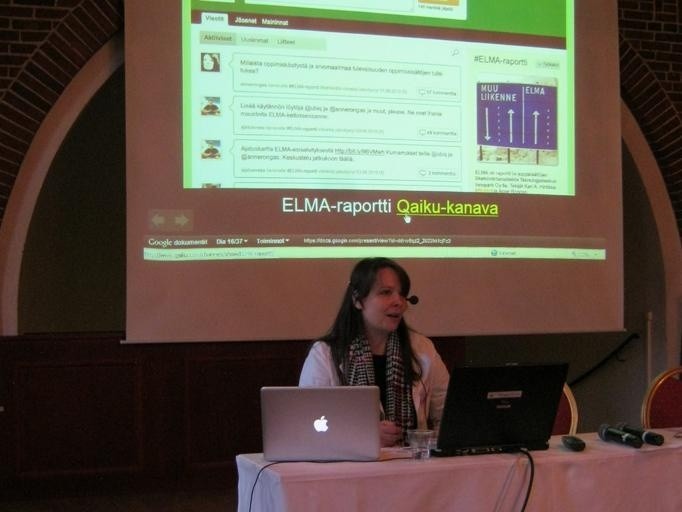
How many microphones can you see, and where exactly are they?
[617,422,664,447]
[406,295,419,305]
[598,424,643,449]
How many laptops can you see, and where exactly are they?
[259,385,384,462]
[403,362,568,458]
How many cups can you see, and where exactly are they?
[406,430,432,459]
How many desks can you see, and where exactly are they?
[234,426,682,512]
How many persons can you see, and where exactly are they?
[299,256,451,448]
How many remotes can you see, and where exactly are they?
[560,434,584,452]
